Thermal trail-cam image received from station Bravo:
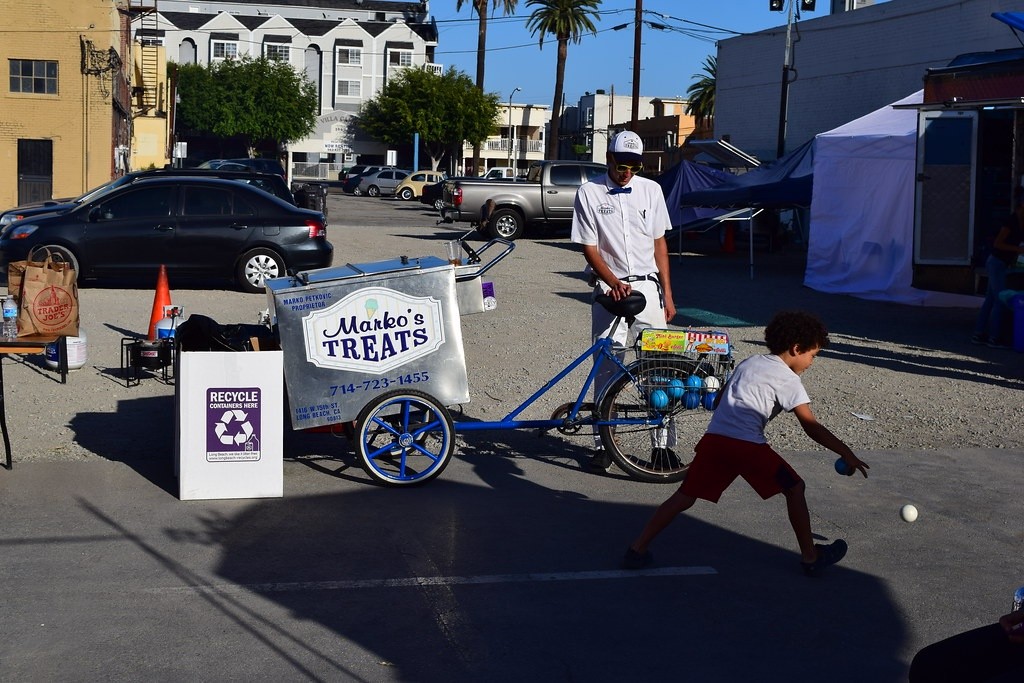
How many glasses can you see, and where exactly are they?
[611,152,643,174]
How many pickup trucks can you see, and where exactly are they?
[436,160,621,240]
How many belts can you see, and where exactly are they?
[622,274,664,309]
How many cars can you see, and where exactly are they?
[0,159,335,292]
[336,163,523,199]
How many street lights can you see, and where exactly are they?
[508,88,521,167]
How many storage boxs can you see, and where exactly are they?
[173,326,283,501]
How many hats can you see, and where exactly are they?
[609,130,643,155]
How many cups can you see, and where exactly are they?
[443,240,463,267]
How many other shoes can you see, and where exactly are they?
[802,537,848,576]
[652,449,684,470]
[590,451,614,473]
[624,547,641,572]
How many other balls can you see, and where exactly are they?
[835,458,849,475]
[901,503,918,523]
[686,375,701,392]
[668,380,684,397]
[682,391,700,409]
[702,392,721,411]
[650,390,668,408]
[704,376,720,393]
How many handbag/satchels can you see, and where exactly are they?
[16,245,80,338]
[7,244,52,299]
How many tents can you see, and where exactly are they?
[657,87,982,306]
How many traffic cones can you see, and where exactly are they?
[148,265,175,342]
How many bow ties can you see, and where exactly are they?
[609,187,632,194]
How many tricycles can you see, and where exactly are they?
[261,236,738,490]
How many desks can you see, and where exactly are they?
[0,335,68,385]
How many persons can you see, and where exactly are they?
[970,184,1024,348]
[571,131,688,473]
[624,312,869,575]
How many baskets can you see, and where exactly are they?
[634,327,730,414]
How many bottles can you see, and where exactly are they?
[1016,253,1024,268]
[3,294,19,337]
